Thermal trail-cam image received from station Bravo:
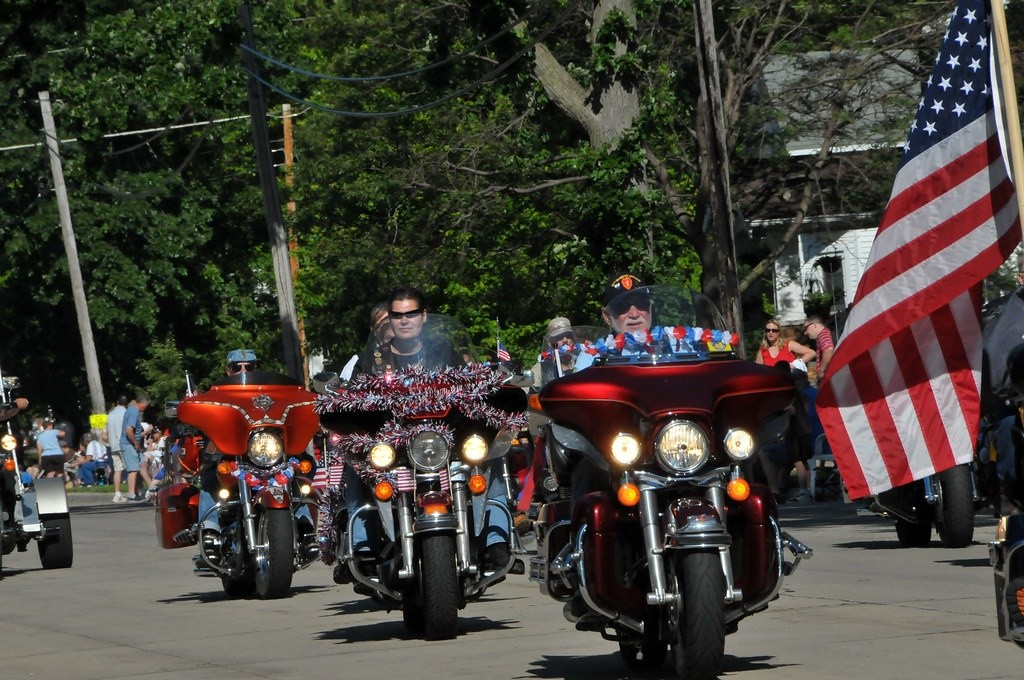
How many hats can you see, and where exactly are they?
[603,273,656,309]
[227,350,258,366]
[547,317,575,339]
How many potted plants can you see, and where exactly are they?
[813,256,844,273]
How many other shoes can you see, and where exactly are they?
[789,494,809,503]
[128,494,148,504]
[113,493,129,504]
[490,543,525,574]
[193,529,221,568]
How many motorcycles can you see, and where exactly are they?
[808,360,1024,649]
[528,283,813,680]
[0,376,73,570]
[154,371,340,600]
[311,314,534,639]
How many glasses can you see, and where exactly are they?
[230,365,257,373]
[611,298,651,315]
[388,308,425,320]
[765,327,779,334]
[804,322,813,333]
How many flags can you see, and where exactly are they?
[498,342,511,361]
[815,0,1024,501]
[310,451,332,488]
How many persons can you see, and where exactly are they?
[35,420,114,489]
[752,317,835,508]
[528,275,661,619]
[185,348,321,564]
[0,397,29,421]
[974,244,1024,510]
[103,383,179,503]
[332,288,525,585]
